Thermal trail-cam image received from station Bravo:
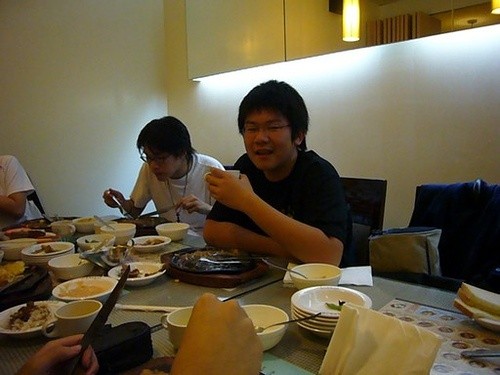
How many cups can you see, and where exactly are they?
[51,220,77,237]
[42,300,105,338]
[203,170,240,200]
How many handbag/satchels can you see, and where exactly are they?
[369,226,442,278]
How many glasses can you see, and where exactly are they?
[140,150,173,162]
[244,122,292,136]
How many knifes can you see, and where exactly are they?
[62,264,131,375]
[139,204,175,219]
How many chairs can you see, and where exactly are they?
[408,179,500,294]
[341,176,388,265]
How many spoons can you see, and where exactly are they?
[254,311,322,332]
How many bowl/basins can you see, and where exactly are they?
[289,263,342,289]
[77,233,117,252]
[52,275,120,302]
[0,238,38,261]
[100,223,136,245]
[48,253,94,280]
[127,235,171,253]
[167,305,195,349]
[72,216,96,233]
[156,223,190,241]
[240,304,290,352]
[108,261,167,287]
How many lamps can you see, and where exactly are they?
[342,0,360,43]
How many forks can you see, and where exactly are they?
[106,190,133,220]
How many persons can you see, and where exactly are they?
[103,116,226,226]
[203,80,354,267]
[0,154,42,231]
[16,293,263,375]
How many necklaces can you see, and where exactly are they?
[167,169,187,222]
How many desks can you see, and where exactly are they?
[0,216,500,375]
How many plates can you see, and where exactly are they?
[1,231,56,239]
[0,301,66,336]
[20,241,75,267]
[290,286,373,336]
